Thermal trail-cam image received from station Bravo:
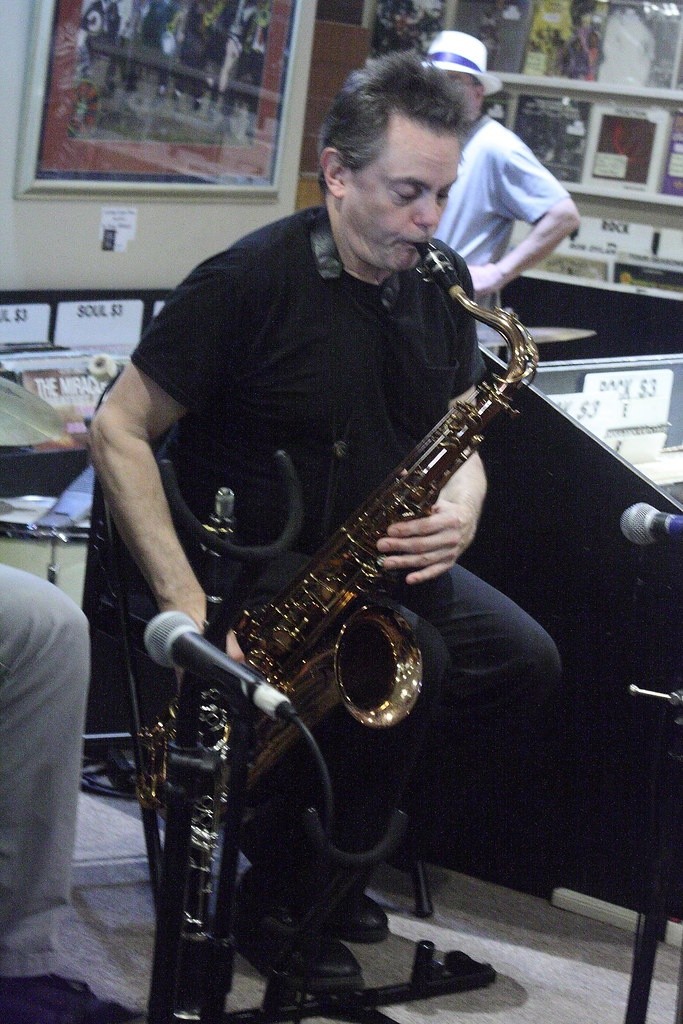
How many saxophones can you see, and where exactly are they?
[139,240,540,820]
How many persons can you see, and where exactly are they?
[422,29,579,349]
[0,563,133,1024]
[88,49,564,990]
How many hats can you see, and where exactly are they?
[422,30,502,94]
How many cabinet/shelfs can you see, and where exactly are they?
[435,0,683,299]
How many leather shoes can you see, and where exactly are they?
[229,912,363,992]
[325,890,384,943]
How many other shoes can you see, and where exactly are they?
[0,971,138,1024]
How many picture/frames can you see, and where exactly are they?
[13,0,320,204]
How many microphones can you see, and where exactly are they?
[619,501,683,546]
[144,610,300,724]
